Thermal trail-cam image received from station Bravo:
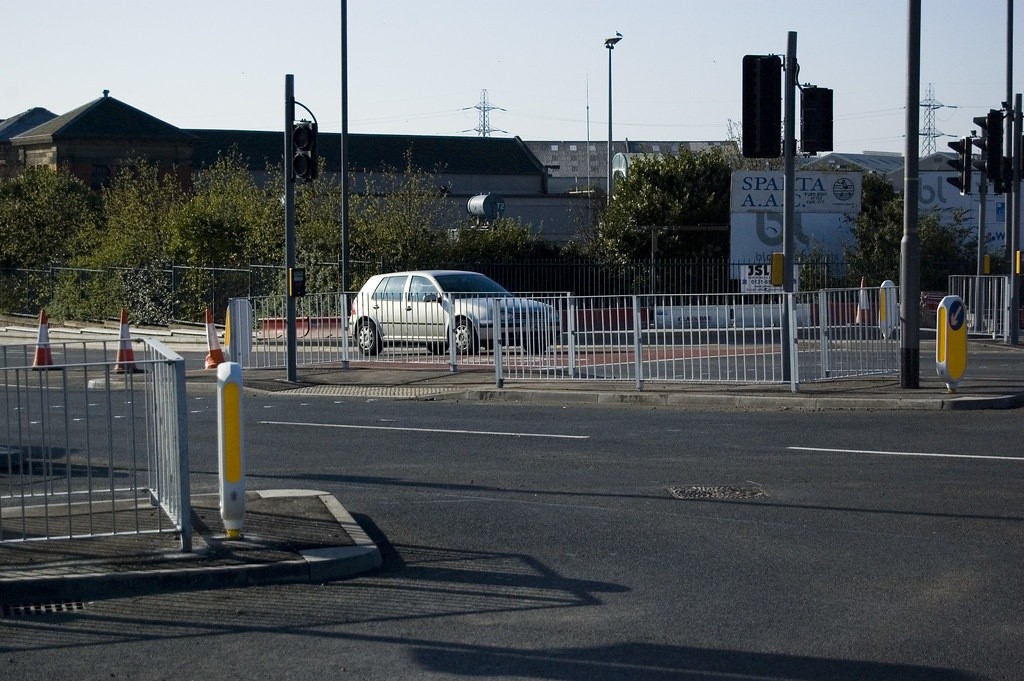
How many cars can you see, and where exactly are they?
[348,270,560,357]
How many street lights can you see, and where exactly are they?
[601,32,623,216]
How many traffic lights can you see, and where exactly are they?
[971,108,1003,179]
[946,135,971,198]
[291,121,318,184]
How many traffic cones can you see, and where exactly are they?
[109,307,148,374]
[28,309,64,371]
[204,305,225,370]
[853,276,872,326]
